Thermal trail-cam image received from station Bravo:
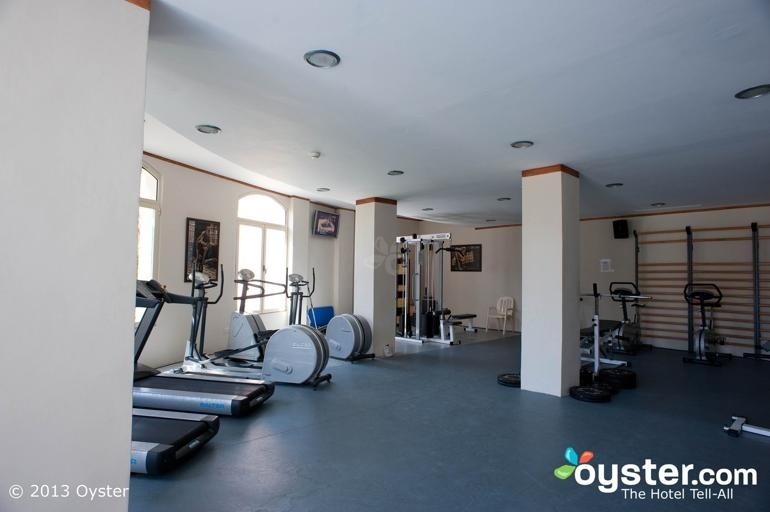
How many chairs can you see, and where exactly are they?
[485,296,515,336]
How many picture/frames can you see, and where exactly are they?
[183,217,220,282]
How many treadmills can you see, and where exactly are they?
[135,279,274,416]
[130,408,220,475]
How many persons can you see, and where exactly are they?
[453,247,467,272]
[192,228,218,267]
